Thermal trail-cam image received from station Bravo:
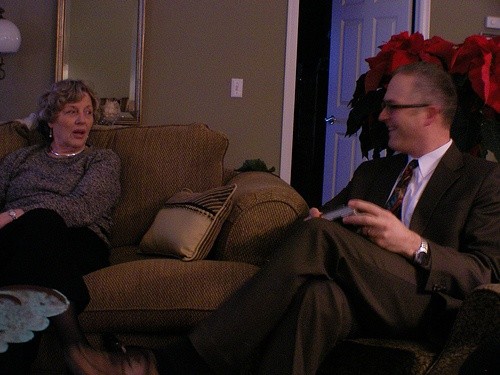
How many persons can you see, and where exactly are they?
[0,78,122,375]
[67,60,500,375]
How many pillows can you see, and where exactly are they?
[135,182,239,263]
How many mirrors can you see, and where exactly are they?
[54,0,146,125]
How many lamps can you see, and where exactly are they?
[0,8,22,80]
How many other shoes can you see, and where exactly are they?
[64,342,158,375]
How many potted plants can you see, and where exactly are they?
[346,32,500,161]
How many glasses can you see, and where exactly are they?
[382,103,432,115]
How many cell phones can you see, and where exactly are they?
[320,207,357,222]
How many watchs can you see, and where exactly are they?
[412,236,429,266]
[7,208,17,221]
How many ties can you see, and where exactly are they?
[385,159,419,222]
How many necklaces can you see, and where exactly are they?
[48,144,85,156]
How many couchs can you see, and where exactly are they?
[0,116,310,375]
[317,284,500,375]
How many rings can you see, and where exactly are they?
[367,227,372,236]
